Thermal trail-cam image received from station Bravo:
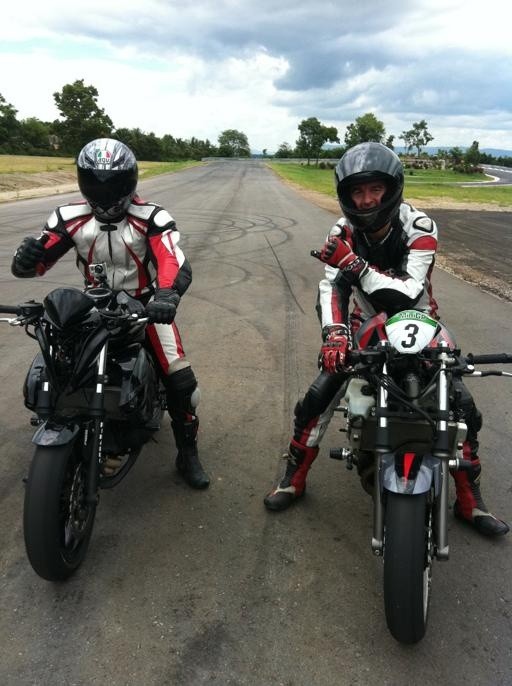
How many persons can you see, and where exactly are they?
[261,139,511,540]
[9,137,214,490]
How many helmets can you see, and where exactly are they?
[77,138,138,222]
[335,142,404,231]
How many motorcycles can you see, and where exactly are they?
[319,309,511,648]
[0,264,174,583]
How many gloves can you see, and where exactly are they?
[145,289,180,325]
[12,234,50,273]
[317,324,352,375]
[309,226,368,279]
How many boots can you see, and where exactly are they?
[264,438,319,512]
[453,460,510,537]
[171,418,211,489]
